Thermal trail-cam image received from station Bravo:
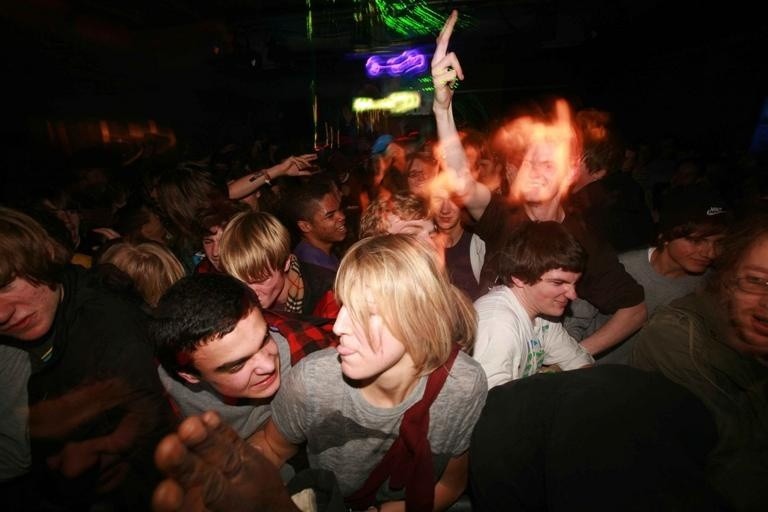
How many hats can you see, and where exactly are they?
[660,183,734,230]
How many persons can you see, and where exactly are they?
[1,9,767,511]
